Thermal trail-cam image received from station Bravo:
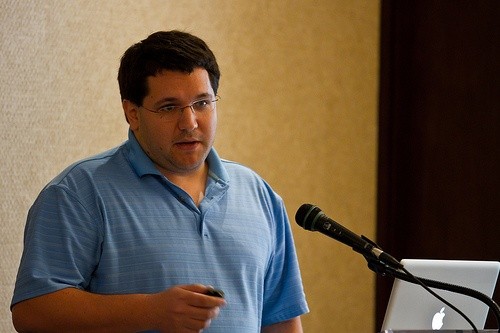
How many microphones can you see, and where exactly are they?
[295,203,403,270]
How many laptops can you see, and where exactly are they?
[382,259,500,331]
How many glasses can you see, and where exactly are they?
[142,94,222,119]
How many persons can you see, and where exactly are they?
[9,30,312,333]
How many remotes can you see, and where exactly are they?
[206,289,224,298]
